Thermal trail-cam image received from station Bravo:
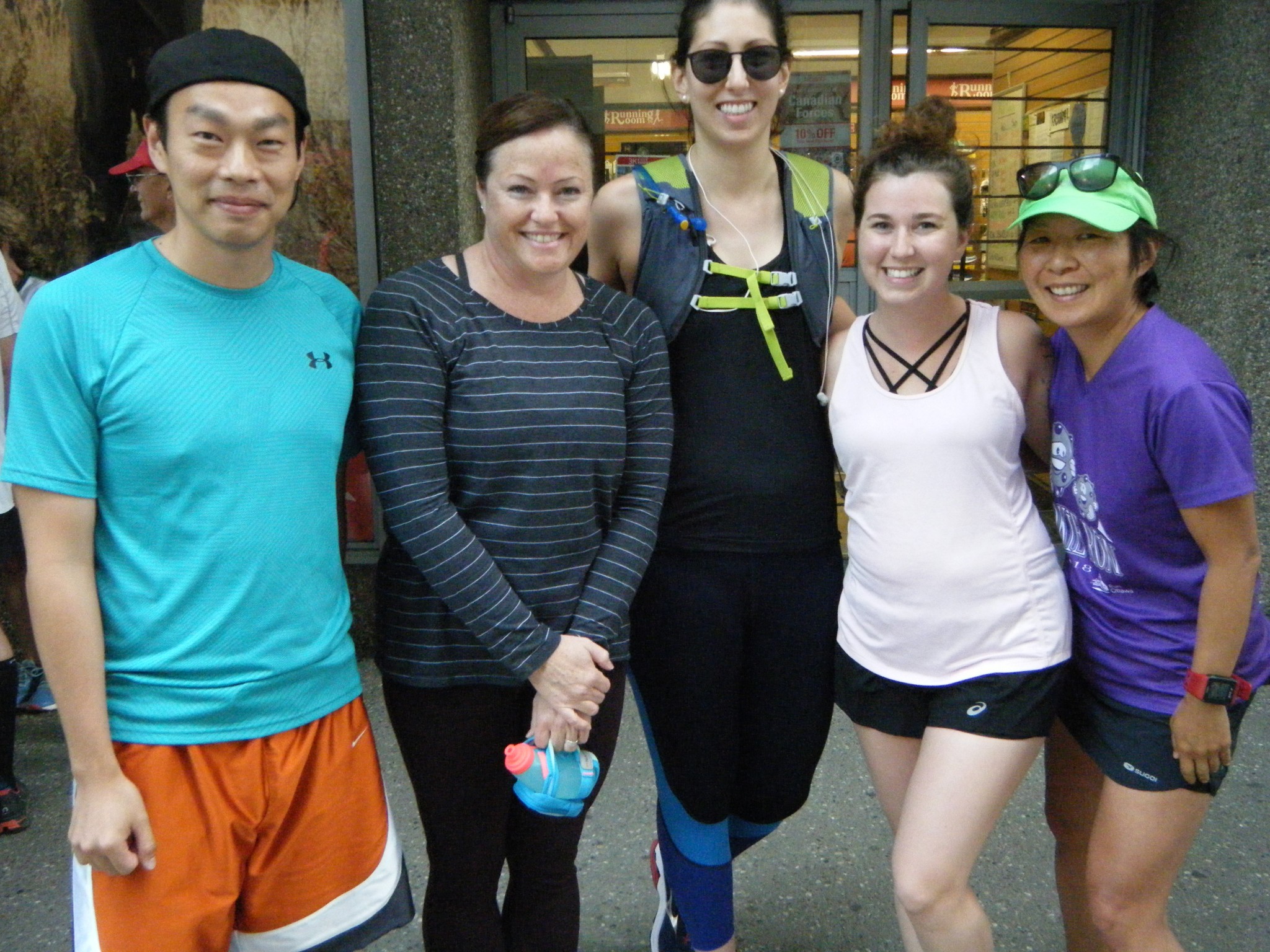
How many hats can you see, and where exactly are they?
[1006,157,1160,232]
[149,25,312,128]
[108,138,155,174]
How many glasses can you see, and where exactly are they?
[125,173,169,184]
[1017,154,1143,207]
[686,44,786,83]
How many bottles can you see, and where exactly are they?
[504,742,600,800]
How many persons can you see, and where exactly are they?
[825,128,1093,952]
[109,125,174,236]
[0,227,60,835]
[1007,149,1270,952]
[589,0,862,951]
[0,25,414,950]
[350,91,658,952]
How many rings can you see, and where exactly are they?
[565,740,579,748]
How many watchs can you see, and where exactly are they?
[1176,669,1254,706]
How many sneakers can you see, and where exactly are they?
[649,839,691,952]
[16,660,36,704]
[27,666,57,711]
[0,780,30,833]
